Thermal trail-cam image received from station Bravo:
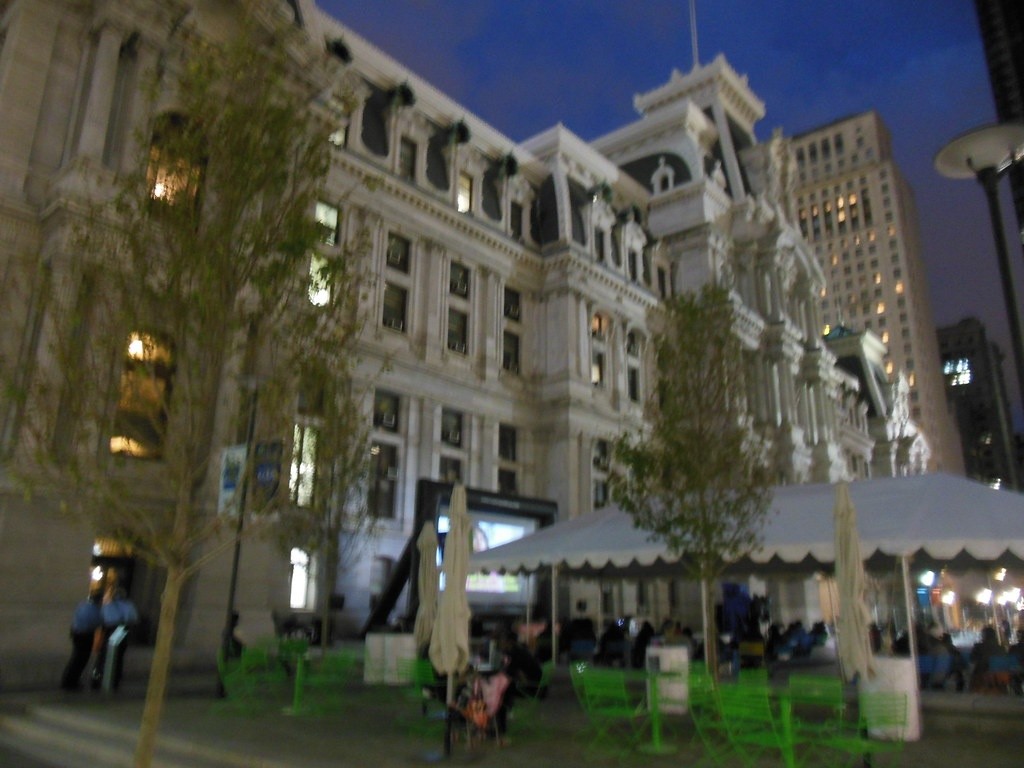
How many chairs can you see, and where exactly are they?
[922,650,1024,695]
[568,664,906,768]
[214,637,552,753]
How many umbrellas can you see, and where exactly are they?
[835,478,877,697]
[414,515,438,652]
[427,479,474,757]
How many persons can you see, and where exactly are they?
[62,583,138,691]
[867,619,1022,695]
[483,629,542,698]
[490,591,827,669]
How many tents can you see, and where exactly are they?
[464,474,1023,686]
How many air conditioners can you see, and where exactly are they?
[382,413,395,427]
[390,319,402,330]
[389,251,401,264]
[450,343,464,353]
[510,305,518,316]
[509,364,517,373]
[449,430,459,442]
[388,466,398,480]
[456,282,467,294]
